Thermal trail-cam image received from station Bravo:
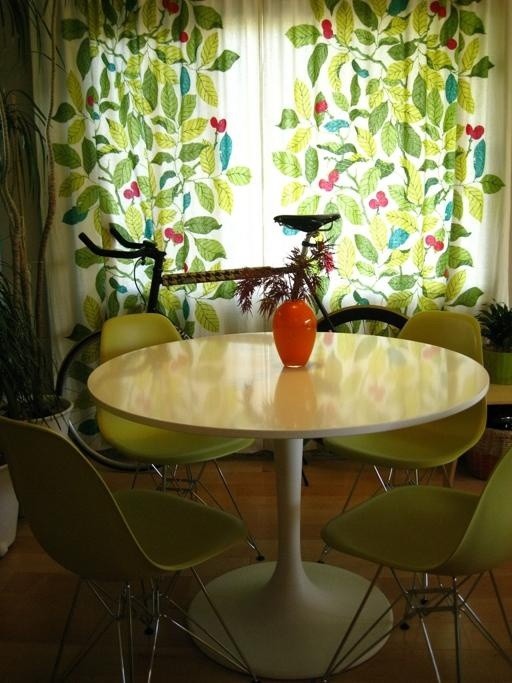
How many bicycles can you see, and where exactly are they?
[56,208,424,473]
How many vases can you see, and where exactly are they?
[272,296,319,370]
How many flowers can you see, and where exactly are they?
[236,230,340,321]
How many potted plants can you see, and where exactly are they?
[471,291,512,388]
[0,254,74,444]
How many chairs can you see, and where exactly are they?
[1,311,512,682]
